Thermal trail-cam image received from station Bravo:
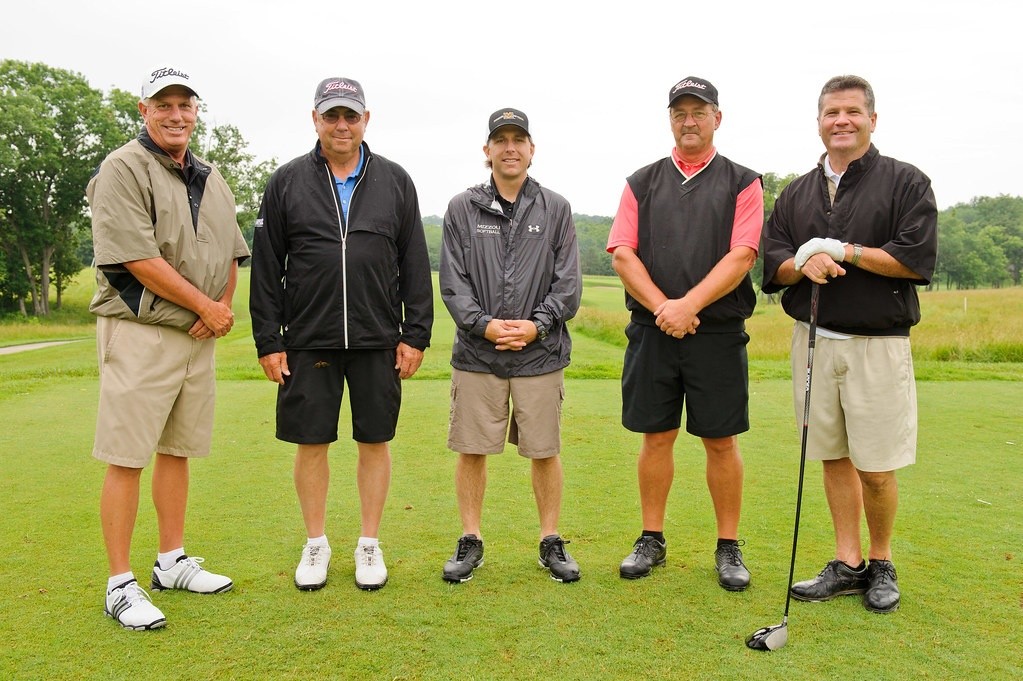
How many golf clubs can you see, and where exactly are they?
[745,282,819,653]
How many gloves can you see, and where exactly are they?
[794,238,848,271]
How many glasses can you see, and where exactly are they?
[321,108,362,125]
[670,108,716,123]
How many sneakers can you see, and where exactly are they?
[864,556,901,614]
[441,534,485,583]
[791,559,868,602]
[538,533,581,582]
[713,539,753,591]
[620,535,667,579]
[354,542,387,589]
[151,555,234,594]
[103,578,166,630]
[294,542,332,590]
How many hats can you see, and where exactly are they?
[141,63,199,100]
[487,108,532,139]
[667,76,719,109]
[314,77,366,116]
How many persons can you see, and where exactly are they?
[86,64,253,630]
[761,75,938,612]
[606,76,764,593]
[248,76,434,591]
[438,107,583,584]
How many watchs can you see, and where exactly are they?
[851,243,863,266]
